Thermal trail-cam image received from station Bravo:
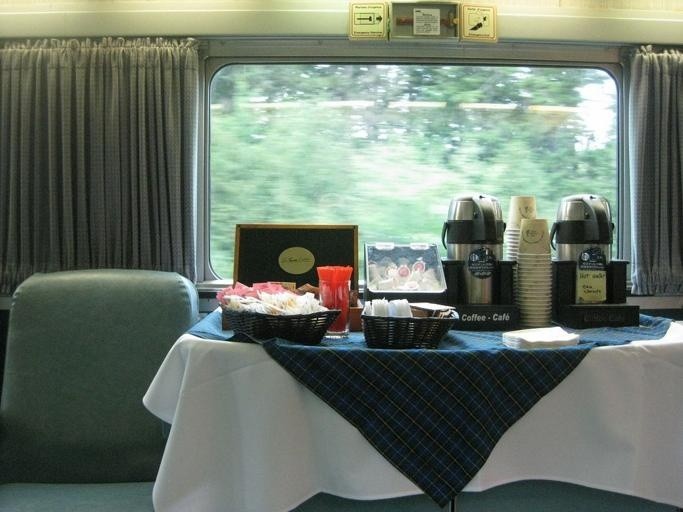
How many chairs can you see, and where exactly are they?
[0,269,198,511]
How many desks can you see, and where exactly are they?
[185,304,682,511]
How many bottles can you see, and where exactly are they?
[550,195,615,310]
[442,193,506,309]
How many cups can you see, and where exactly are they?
[502,195,552,331]
[318,279,352,345]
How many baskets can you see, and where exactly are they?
[360,307,460,348]
[220,305,342,345]
